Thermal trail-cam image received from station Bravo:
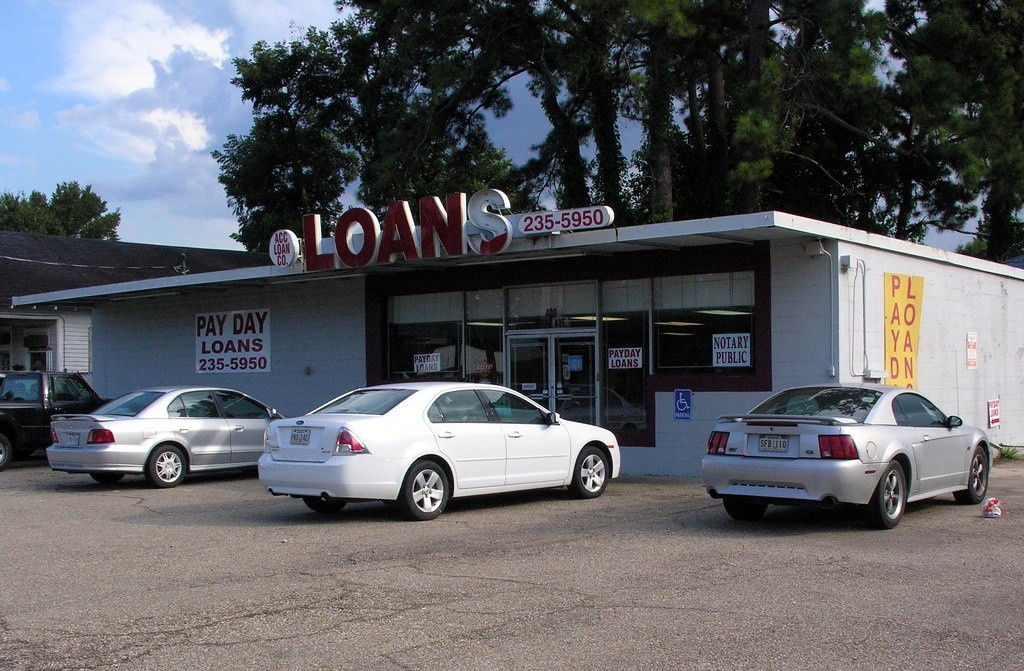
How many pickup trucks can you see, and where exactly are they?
[0,371,118,470]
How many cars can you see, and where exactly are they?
[45,385,289,487]
[700,381,993,528]
[492,382,648,440]
[256,379,621,520]
